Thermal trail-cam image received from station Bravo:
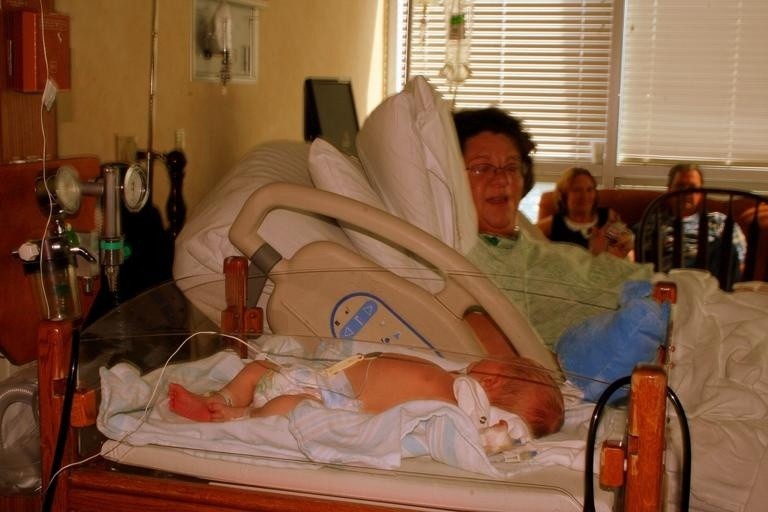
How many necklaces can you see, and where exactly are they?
[481,217,524,234]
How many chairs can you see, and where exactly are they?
[635,187,767,290]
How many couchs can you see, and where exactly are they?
[539,186,767,284]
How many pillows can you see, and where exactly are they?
[304,76,481,291]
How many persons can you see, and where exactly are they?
[627,162,748,277]
[533,164,636,263]
[435,101,540,238]
[162,329,564,458]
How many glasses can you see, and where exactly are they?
[465,162,529,185]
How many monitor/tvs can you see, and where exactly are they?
[303,78,360,143]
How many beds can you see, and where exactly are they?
[37,316,670,512]
[170,129,768,512]
